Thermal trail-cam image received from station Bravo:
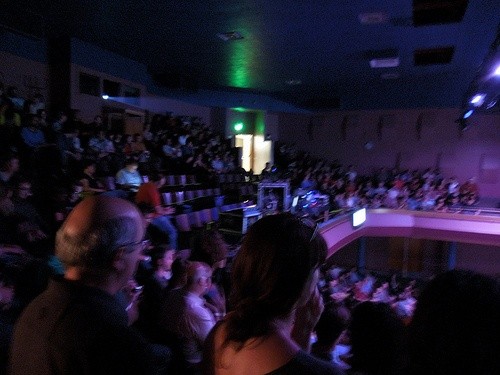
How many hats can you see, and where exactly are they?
[188,230,227,263]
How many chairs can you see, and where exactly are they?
[161,173,257,233]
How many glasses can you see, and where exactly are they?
[299,216,318,245]
[118,239,151,251]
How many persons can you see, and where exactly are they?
[9,196,144,375]
[0,98,20,129]
[54,181,82,226]
[345,299,409,374]
[30,109,48,130]
[0,151,48,243]
[136,173,178,250]
[156,263,219,375]
[51,106,156,160]
[408,268,500,375]
[19,114,56,159]
[156,111,481,218]
[201,213,346,374]
[117,158,145,196]
[28,93,45,113]
[79,159,129,199]
[8,86,25,113]
[305,305,349,367]
[315,257,421,319]
[111,202,226,326]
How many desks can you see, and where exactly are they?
[218,211,265,235]
